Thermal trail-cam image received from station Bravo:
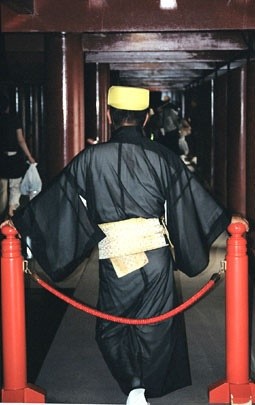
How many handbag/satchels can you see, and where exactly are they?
[20,161,41,202]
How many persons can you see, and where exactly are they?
[0,84,249,405]
[146,95,206,180]
[0,95,35,221]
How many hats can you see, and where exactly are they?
[107,85,150,111]
